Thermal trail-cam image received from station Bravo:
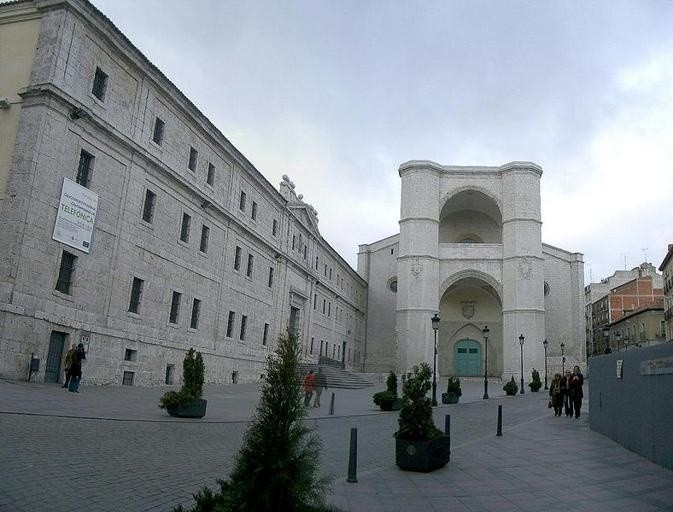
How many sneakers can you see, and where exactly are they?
[61,385,81,393]
[566,408,573,418]
[313,403,321,408]
[575,412,580,420]
[303,404,311,409]
[554,411,562,417]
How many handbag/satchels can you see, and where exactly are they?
[548,400,552,408]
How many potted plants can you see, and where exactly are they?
[372,362,462,473]
[503,368,542,398]
[165,348,207,419]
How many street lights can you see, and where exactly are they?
[602,327,629,354]
[560,342,565,376]
[430,313,440,407]
[518,334,525,394]
[482,325,490,399]
[542,339,549,390]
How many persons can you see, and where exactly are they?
[549,372,563,416]
[560,369,572,417]
[303,369,313,408]
[61,343,77,389]
[569,365,583,419]
[67,342,87,393]
[311,367,327,409]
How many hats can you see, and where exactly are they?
[77,344,83,348]
